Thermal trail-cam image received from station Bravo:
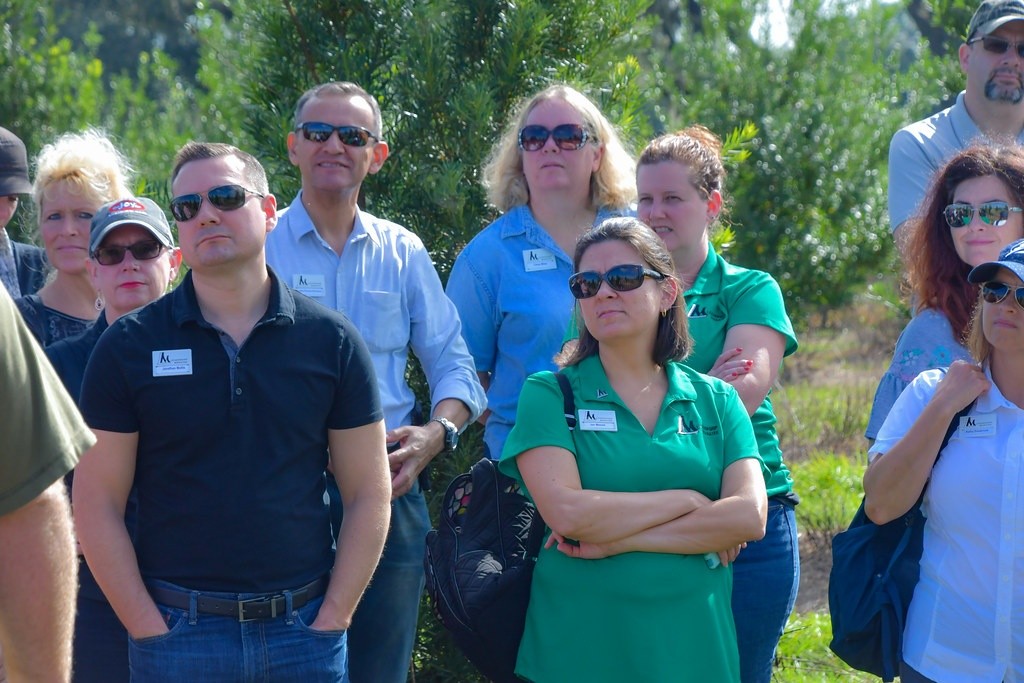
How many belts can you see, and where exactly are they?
[146,574,324,622]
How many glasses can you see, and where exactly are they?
[295,122,379,146]
[170,185,264,222]
[968,36,1024,58]
[568,265,664,299]
[982,283,1024,310]
[942,202,1023,229]
[93,240,163,265]
[519,125,598,153]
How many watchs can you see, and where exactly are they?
[425,417,459,453]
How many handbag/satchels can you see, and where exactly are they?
[828,366,975,682]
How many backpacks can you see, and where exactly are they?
[422,371,577,682]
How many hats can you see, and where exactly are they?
[967,238,1024,284]
[88,197,174,259]
[0,127,33,197]
[967,0,1024,43]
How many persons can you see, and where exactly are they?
[47,198,181,683]
[265,82,488,683]
[864,237,1024,683]
[73,143,391,682]
[562,125,800,683]
[581,280,589,297]
[446,86,643,461]
[864,141,1024,443]
[887,0,1024,245]
[0,282,97,683]
[529,136,540,150]
[498,216,772,683]
[174,201,192,219]
[15,129,125,349]
[0,127,51,299]
